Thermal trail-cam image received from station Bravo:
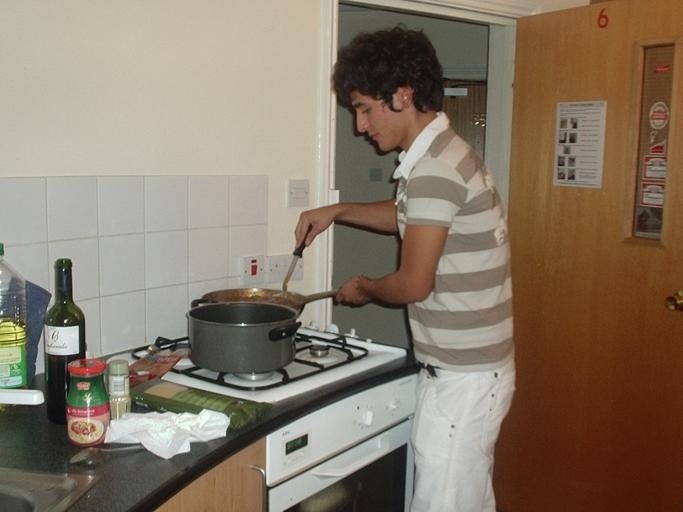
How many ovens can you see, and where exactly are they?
[268,364,421,512]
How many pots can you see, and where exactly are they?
[186,299,302,373]
[202,287,336,319]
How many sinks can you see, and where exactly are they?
[0,466,104,512]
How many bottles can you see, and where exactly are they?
[43,259,87,424]
[107,360,132,419]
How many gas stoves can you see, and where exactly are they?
[132,335,370,390]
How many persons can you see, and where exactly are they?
[293,22,519,512]
